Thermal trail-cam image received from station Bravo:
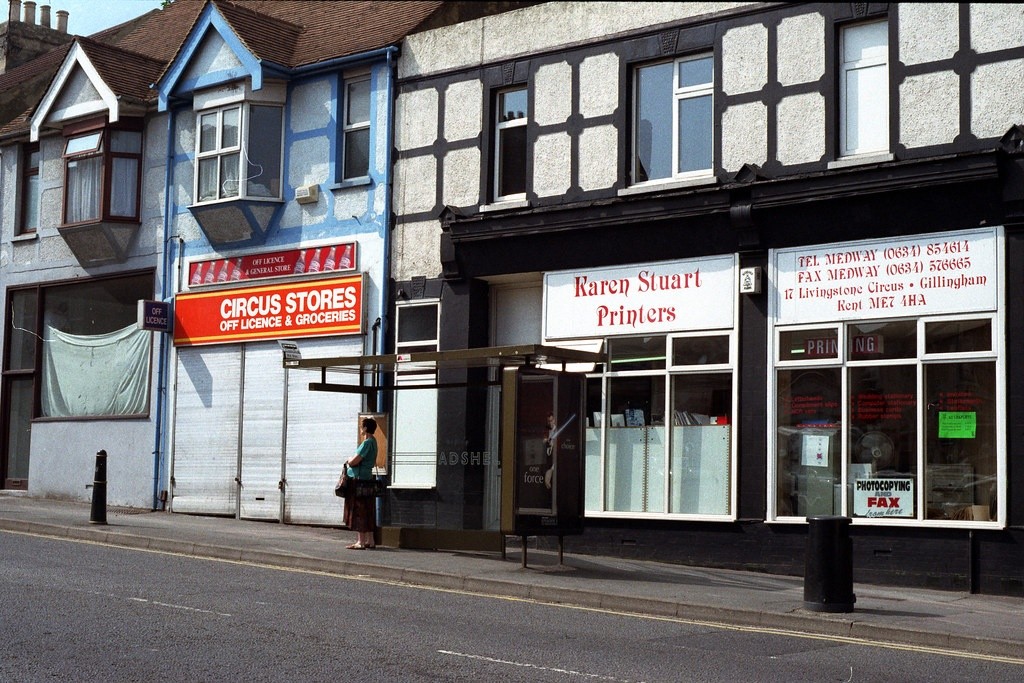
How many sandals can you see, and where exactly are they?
[345,541,375,550]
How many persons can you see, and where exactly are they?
[541,413,560,499]
[347,418,378,550]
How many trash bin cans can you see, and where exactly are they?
[805,516,857,612]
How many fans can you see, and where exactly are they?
[856,431,894,472]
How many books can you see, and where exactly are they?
[673,410,709,425]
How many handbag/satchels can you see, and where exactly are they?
[356,477,387,499]
[333,460,354,500]
